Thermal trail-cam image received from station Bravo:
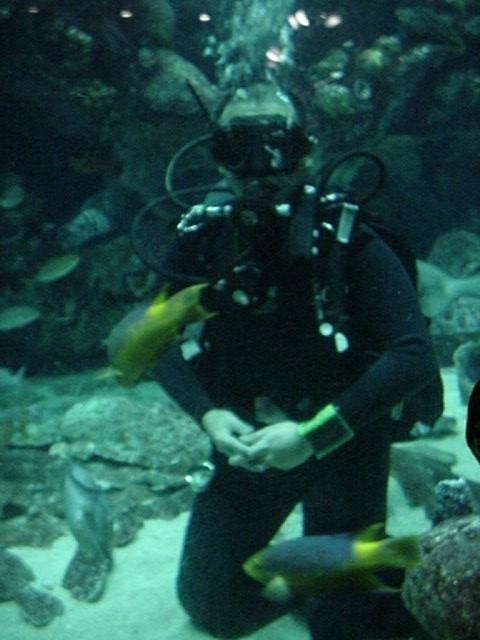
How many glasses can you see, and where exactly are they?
[214,113,313,177]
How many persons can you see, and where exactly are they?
[148,80,444,640]
